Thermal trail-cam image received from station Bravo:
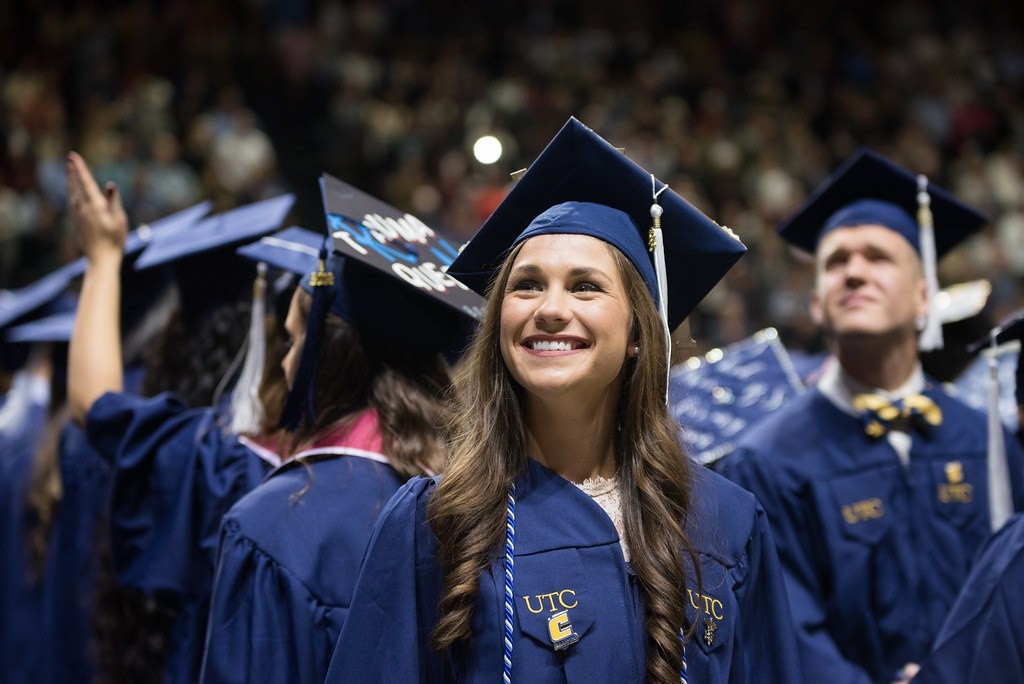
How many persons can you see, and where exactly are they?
[713,144,1024,684]
[200,171,491,684]
[0,192,1023,684]
[324,117,804,684]
[1,0,1023,352]
[62,154,331,684]
[909,315,1023,684]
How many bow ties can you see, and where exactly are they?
[852,391,946,443]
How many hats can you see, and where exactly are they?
[446,113,751,404]
[967,314,1024,529]
[229,225,328,434]
[655,324,808,467]
[774,149,993,351]
[7,313,78,358]
[1,251,96,319]
[277,172,486,431]
[133,193,297,312]
